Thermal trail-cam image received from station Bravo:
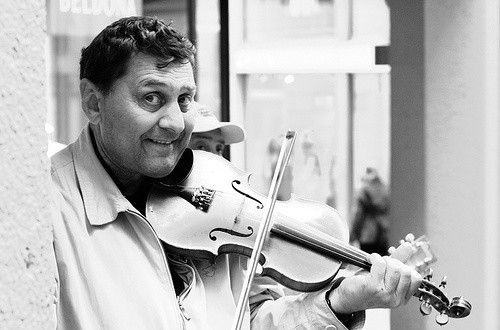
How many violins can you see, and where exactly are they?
[146,147,472,325]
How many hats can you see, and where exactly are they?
[192,101,245,145]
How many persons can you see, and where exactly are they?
[192,102,225,159]
[349,164,393,257]
[50,16,424,330]
